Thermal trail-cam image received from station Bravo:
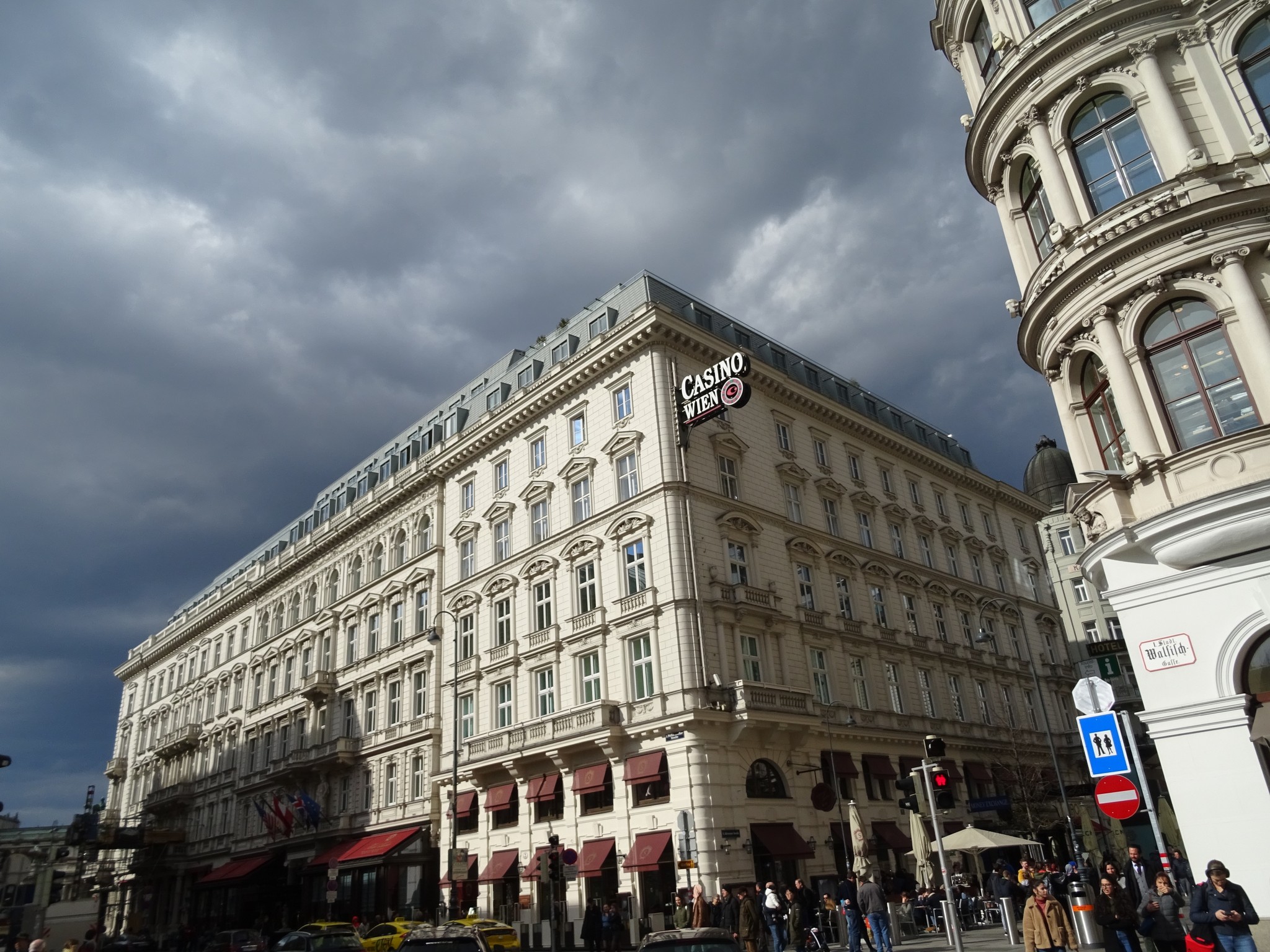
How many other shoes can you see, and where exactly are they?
[1185,892,1188,897]
[773,919,778,923]
[1005,932,1008,936]
[870,948,877,952]
[1017,930,1024,936]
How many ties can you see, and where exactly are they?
[1134,864,1141,875]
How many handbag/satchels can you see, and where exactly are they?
[620,924,625,931]
[1139,893,1154,937]
[1185,934,1215,952]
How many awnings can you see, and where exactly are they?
[822,754,1113,855]
[191,854,274,890]
[752,827,815,859]
[296,827,426,875]
[436,750,673,890]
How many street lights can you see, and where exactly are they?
[825,700,858,875]
[977,598,1088,881]
[428,609,460,922]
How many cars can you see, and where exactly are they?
[440,914,521,952]
[358,916,434,952]
[385,924,493,952]
[267,929,367,952]
[200,929,270,952]
[292,921,361,946]
[101,933,158,952]
[634,927,745,952]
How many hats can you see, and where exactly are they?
[1069,861,1077,867]
[737,887,748,894]
[768,885,776,890]
[722,885,733,894]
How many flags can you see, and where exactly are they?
[253,791,322,841]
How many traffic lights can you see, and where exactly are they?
[548,834,559,845]
[549,851,560,881]
[930,768,956,810]
[925,735,948,756]
[535,852,550,884]
[895,771,926,814]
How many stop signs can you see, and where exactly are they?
[1094,773,1140,818]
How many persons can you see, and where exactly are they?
[1167,845,1196,897]
[835,871,893,952]
[249,898,460,937]
[579,898,628,952]
[881,857,1025,936]
[673,879,837,952]
[14,921,223,952]
[1190,859,1259,952]
[1136,872,1184,952]
[1017,843,1156,952]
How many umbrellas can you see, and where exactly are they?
[909,809,933,888]
[849,800,875,891]
[1078,794,1187,865]
[904,824,1044,897]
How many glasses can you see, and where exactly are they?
[1099,884,1113,889]
[1212,878,1226,883]
